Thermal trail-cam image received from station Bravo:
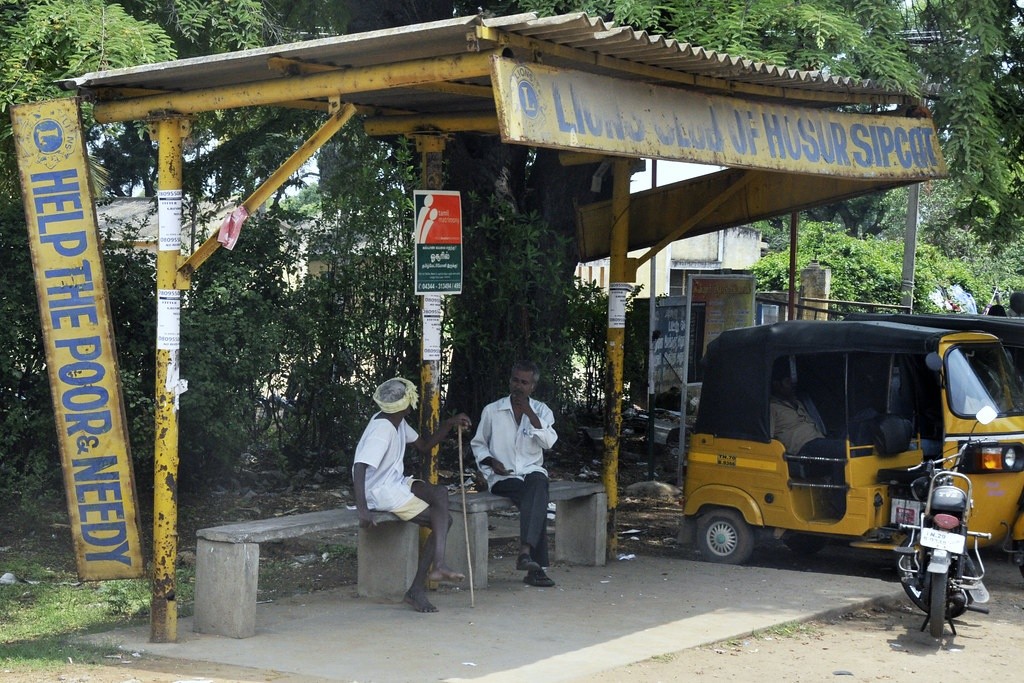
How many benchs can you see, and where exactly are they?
[191,507,422,641]
[439,479,610,590]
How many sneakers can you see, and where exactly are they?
[516,553,539,570]
[523,567,555,587]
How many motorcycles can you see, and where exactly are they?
[893,439,993,640]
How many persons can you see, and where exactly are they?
[1006,293,1024,372]
[351,376,477,613]
[984,305,1013,367]
[471,358,564,589]
[768,372,857,521]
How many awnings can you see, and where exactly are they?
[66,8,940,646]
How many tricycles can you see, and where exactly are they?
[677,321,1024,582]
